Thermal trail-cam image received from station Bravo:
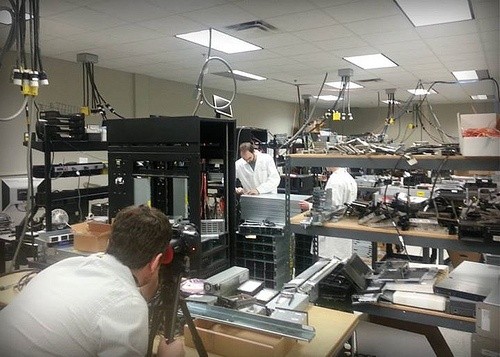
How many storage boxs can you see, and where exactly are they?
[183,316,298,357]
[69,219,113,252]
[456,112,500,157]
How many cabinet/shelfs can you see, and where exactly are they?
[23,127,110,232]
[283,151,500,334]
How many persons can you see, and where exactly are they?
[234,142,280,196]
[297,167,357,212]
[0,204,185,357]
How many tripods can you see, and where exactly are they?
[145,244,208,357]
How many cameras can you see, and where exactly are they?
[166,214,200,262]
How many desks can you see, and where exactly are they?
[150,301,361,357]
